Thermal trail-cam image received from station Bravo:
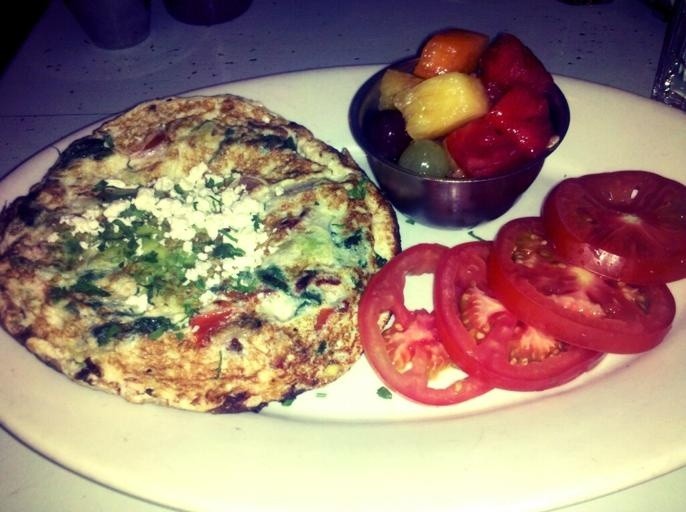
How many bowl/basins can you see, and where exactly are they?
[345,56,572,231]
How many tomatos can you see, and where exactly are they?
[358,170,685,406]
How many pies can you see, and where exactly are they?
[7,95,406,414]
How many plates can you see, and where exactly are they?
[1,62,686,512]
